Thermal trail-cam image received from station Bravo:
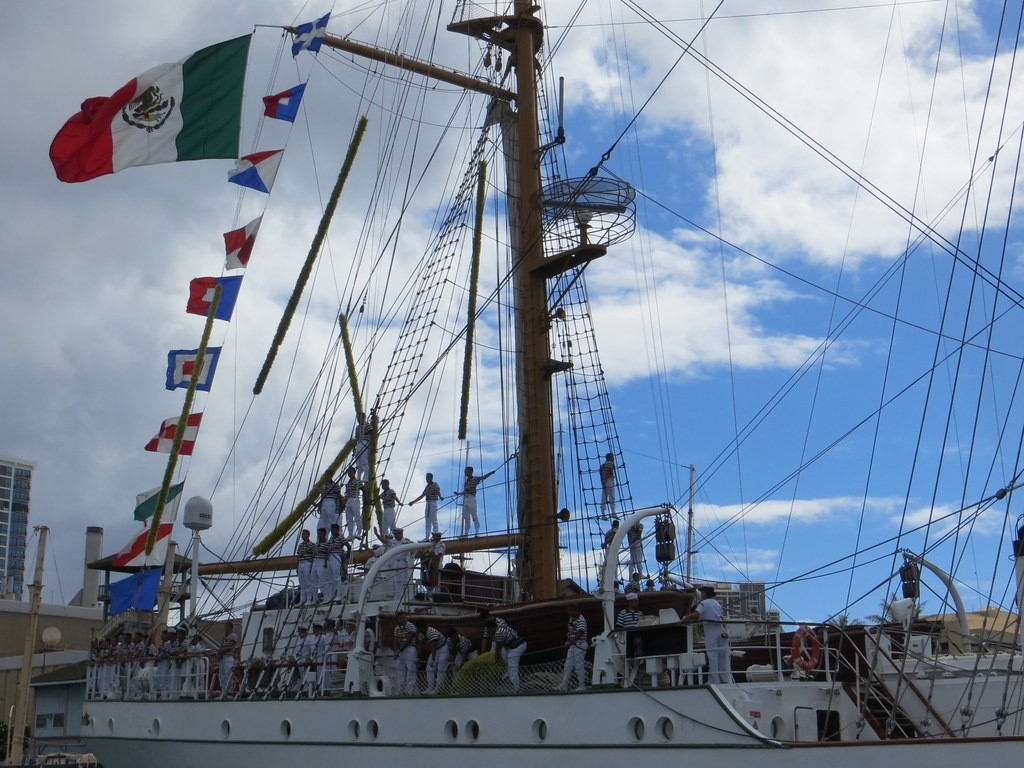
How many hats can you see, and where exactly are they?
[625,592,638,601]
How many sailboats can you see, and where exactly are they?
[75,0,1023,768]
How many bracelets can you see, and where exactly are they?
[400,648,403,651]
[683,616,687,621]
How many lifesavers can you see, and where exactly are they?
[790,624,821,670]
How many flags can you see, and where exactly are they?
[108,214,263,616]
[292,12,331,58]
[228,148,285,194]
[49,34,252,183]
[262,83,306,124]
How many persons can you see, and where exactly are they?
[393,608,527,697]
[366,526,446,601]
[454,466,496,539]
[354,412,374,482]
[599,453,622,521]
[627,520,648,582]
[602,520,619,585]
[316,467,367,542]
[88,628,205,700]
[615,592,643,629]
[553,604,588,692]
[409,473,444,542]
[613,573,657,595]
[216,622,239,699]
[294,617,375,697]
[680,586,733,684]
[372,479,403,540]
[294,524,346,607]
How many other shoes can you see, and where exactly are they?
[93,690,237,703]
[420,687,442,696]
[571,685,587,693]
[391,688,415,696]
[551,682,567,693]
[295,596,345,608]
